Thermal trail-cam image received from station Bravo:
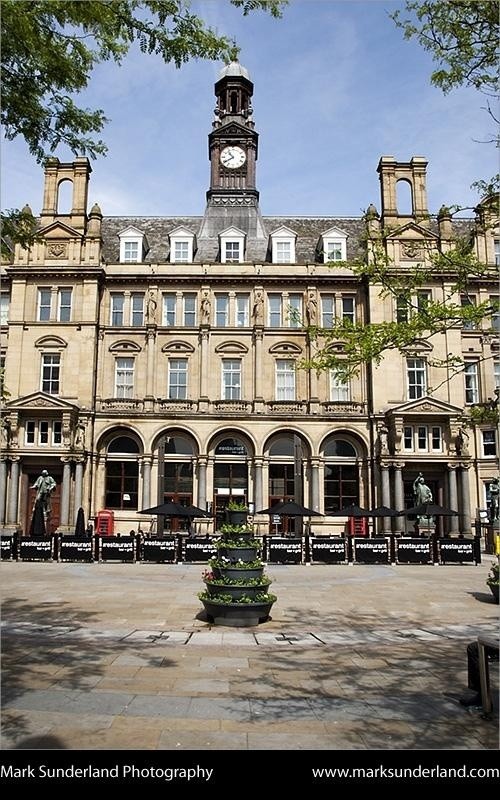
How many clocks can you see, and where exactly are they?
[220,141,249,172]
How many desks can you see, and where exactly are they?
[2,530,486,569]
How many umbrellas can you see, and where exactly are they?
[256,499,288,534]
[29,500,47,536]
[370,506,399,537]
[325,501,377,538]
[137,497,204,539]
[259,498,323,539]
[185,504,222,526]
[399,498,465,538]
[74,507,86,537]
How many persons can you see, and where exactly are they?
[412,471,433,519]
[489,476,500,521]
[459,639,499,712]
[253,292,264,325]
[29,469,57,512]
[307,293,318,325]
[200,292,211,324]
[145,291,157,323]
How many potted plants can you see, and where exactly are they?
[196,499,278,626]
